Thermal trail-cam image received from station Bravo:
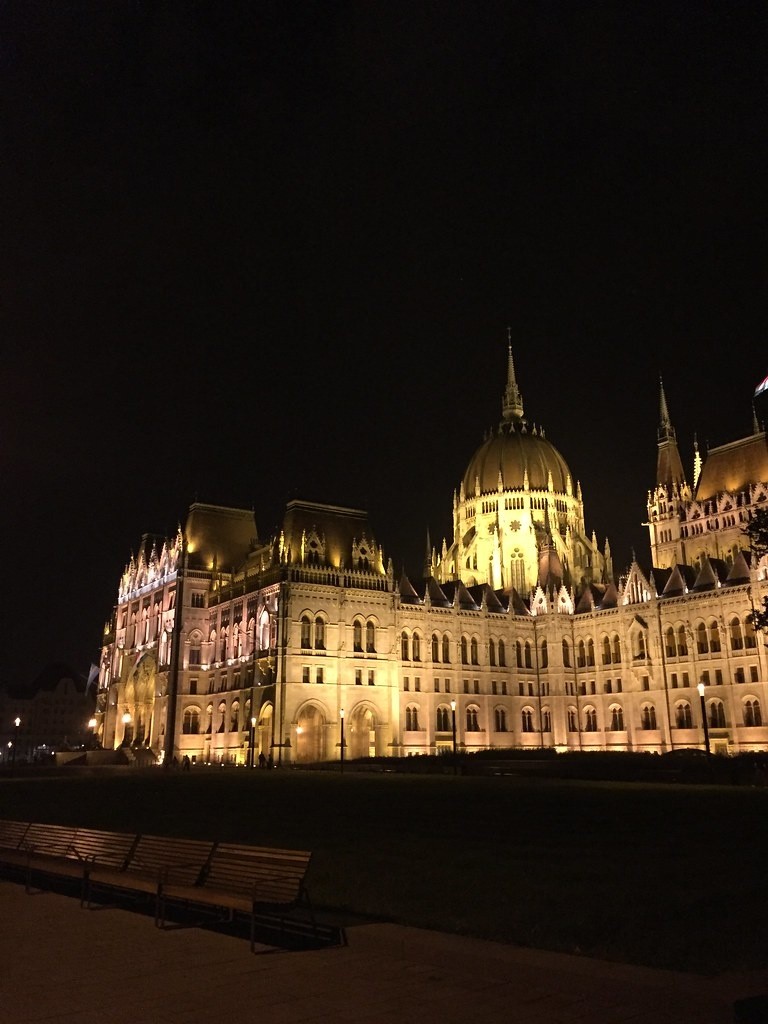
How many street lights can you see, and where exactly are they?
[697,683,711,753]
[450,699,457,753]
[340,709,345,775]
[6,741,12,775]
[123,708,130,747]
[250,718,256,769]
[10,718,20,776]
